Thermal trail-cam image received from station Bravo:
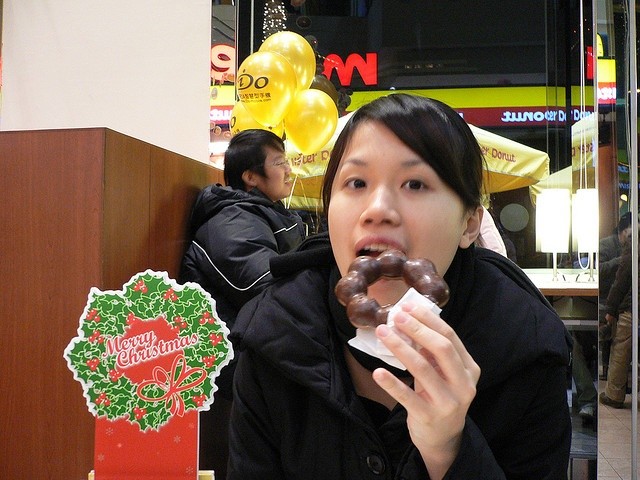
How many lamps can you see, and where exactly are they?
[571,187,599,284]
[534,188,571,283]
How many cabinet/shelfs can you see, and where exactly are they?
[528,280,598,333]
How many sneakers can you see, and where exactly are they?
[599,392,624,409]
[579,404,595,420]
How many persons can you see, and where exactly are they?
[177,128,305,480]
[599,208,640,408]
[224,94,573,479]
[600,211,632,380]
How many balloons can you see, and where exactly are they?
[284,91,338,156]
[309,75,338,106]
[237,50,297,128]
[229,100,284,141]
[259,31,316,90]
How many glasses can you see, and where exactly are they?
[250,156,289,170]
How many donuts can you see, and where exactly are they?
[333,248,450,328]
